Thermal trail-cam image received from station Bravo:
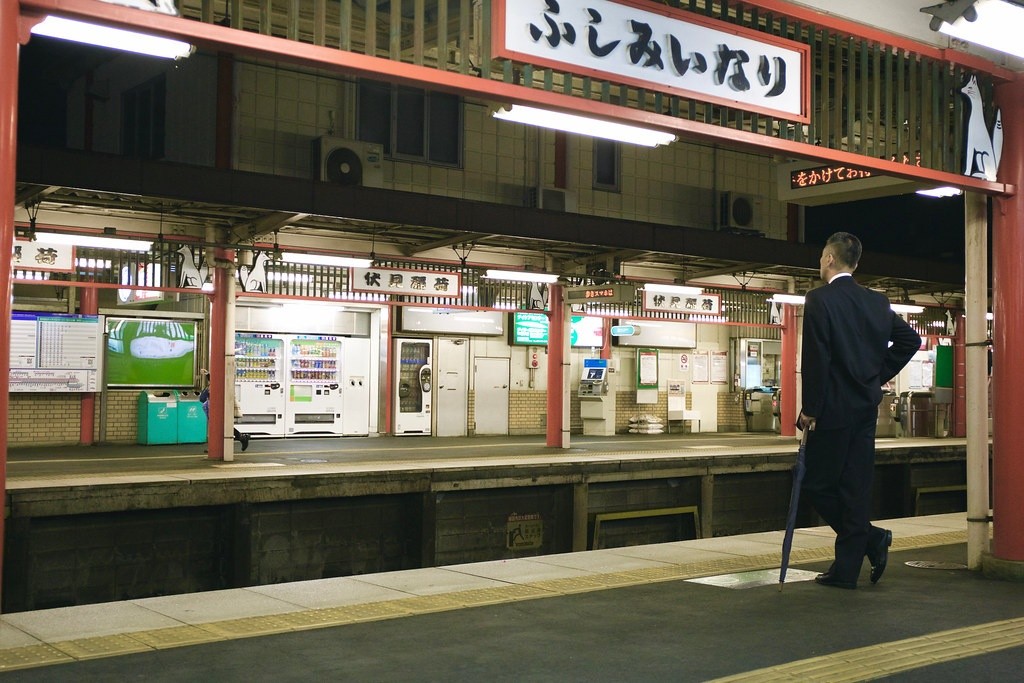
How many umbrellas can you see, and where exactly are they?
[778,420,816,592]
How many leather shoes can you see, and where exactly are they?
[815,572,857,589]
[871,529,892,582]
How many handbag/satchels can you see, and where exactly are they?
[200,385,209,402]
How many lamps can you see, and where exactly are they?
[485,100,679,148]
[29,14,195,61]
[917,1,1024,55]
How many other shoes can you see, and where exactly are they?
[241,434,249,451]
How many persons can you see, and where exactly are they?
[797,232,921,590]
[200,369,250,453]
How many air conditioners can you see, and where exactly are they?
[716,192,765,233]
[314,137,384,188]
[533,187,576,214]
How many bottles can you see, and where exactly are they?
[399,346,429,412]
[234,339,337,380]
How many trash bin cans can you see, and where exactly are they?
[174,390,207,443]
[137,390,178,445]
